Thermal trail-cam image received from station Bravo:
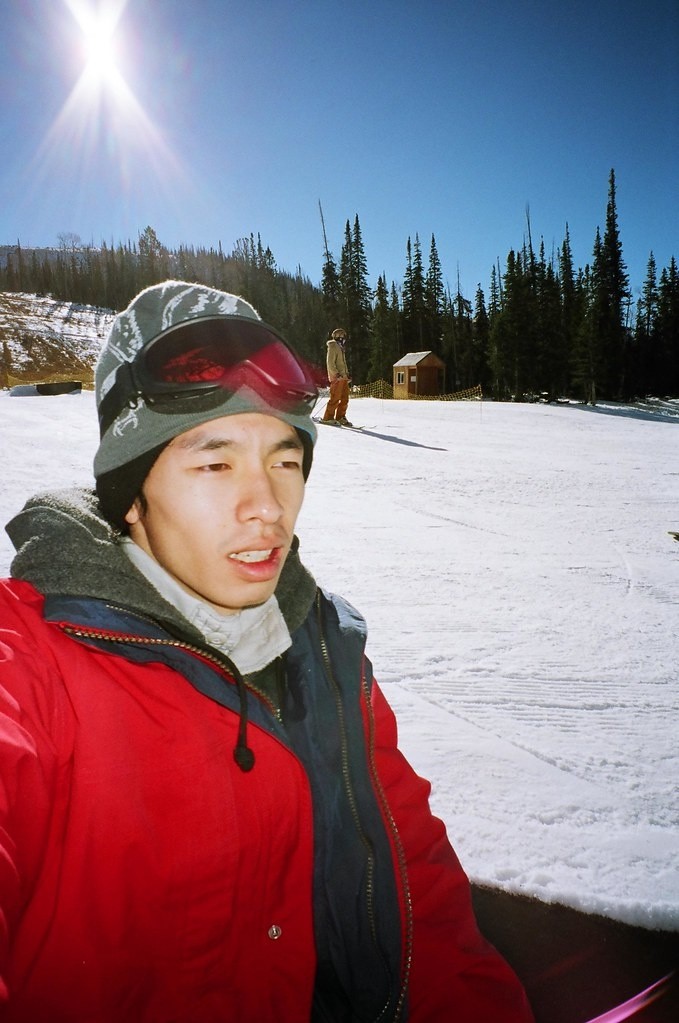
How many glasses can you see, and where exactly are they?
[98,314,316,441]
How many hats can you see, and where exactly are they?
[93,280,318,476]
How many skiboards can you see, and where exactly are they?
[311,417,353,428]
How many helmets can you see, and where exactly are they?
[332,328,347,339]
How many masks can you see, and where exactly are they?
[342,339,346,345]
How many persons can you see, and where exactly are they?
[323,328,352,424]
[0,280,533,1023]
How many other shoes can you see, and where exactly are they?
[323,420,337,424]
[338,417,348,424]
